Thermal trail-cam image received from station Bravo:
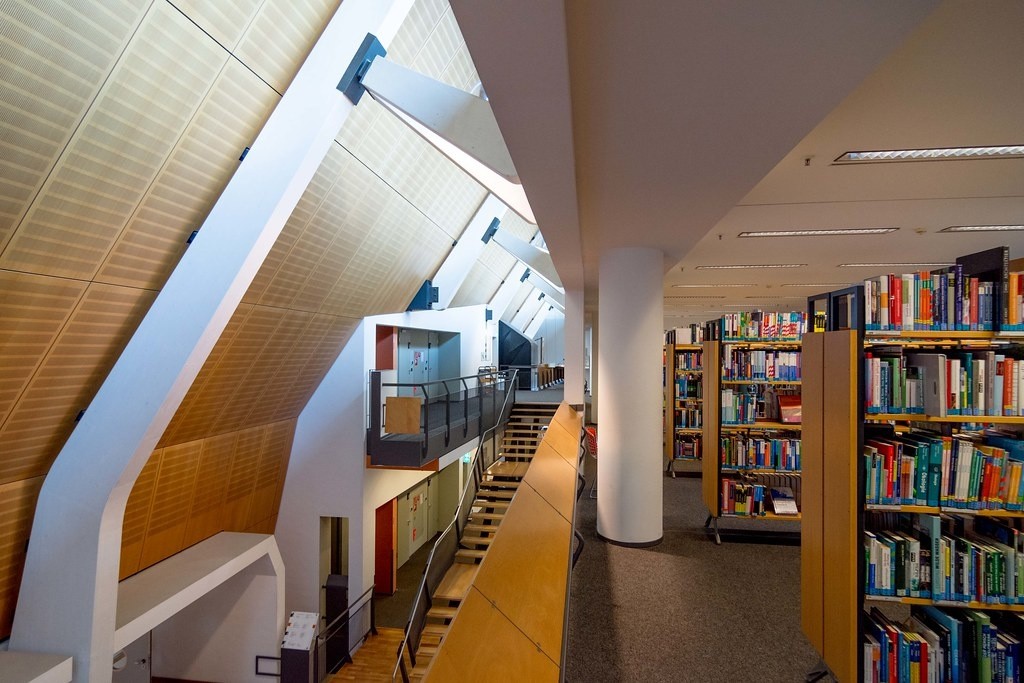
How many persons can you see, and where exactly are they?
[536,426,549,446]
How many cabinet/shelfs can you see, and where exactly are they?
[665,330,703,477]
[478,366,497,397]
[702,319,802,544]
[800,285,1024,683]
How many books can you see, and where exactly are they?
[715,308,827,342]
[676,321,707,347]
[865,513,1024,604]
[864,604,1024,683]
[721,428,801,473]
[865,345,1024,418]
[721,345,801,381]
[666,330,675,344]
[865,419,1024,512]
[663,349,666,433]
[675,351,703,460]
[721,384,801,424]
[721,472,802,517]
[839,246,1024,332]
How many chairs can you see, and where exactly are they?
[584,427,598,499]
